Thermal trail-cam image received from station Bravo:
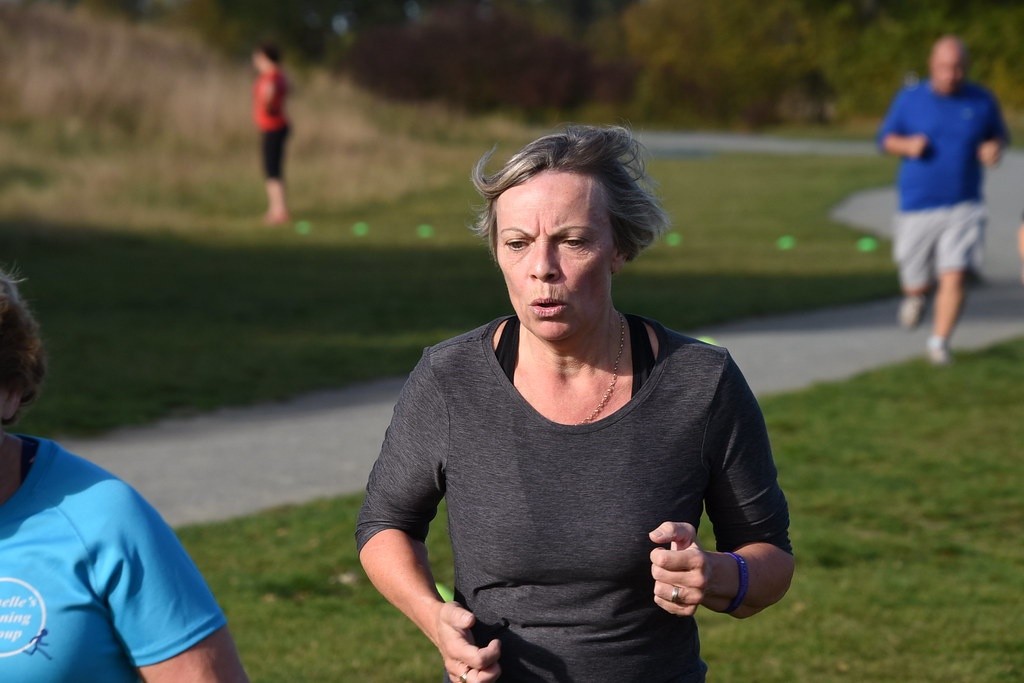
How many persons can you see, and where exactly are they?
[251,40,293,221]
[874,36,1007,364]
[352,118,796,683]
[0,271,252,682]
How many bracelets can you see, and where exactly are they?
[719,551,749,613]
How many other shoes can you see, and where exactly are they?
[925,339,950,364]
[899,297,926,327]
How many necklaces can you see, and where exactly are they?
[572,311,626,426]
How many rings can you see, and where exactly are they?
[671,587,679,603]
